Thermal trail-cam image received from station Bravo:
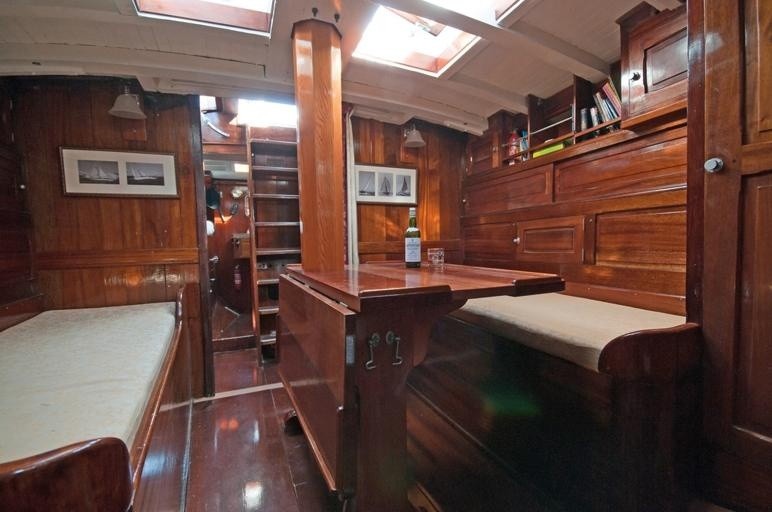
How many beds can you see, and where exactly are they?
[0,284,189,512]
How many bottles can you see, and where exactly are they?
[405,207,421,267]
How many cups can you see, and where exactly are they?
[426,248,444,273]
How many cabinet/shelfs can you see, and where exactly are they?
[460,0,688,316]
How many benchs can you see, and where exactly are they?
[446,291,703,510]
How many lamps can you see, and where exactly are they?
[403,118,427,149]
[107,79,148,120]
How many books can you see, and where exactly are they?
[577,75,624,144]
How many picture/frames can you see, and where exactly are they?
[354,163,419,206]
[58,146,180,200]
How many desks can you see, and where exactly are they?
[277,262,564,512]
